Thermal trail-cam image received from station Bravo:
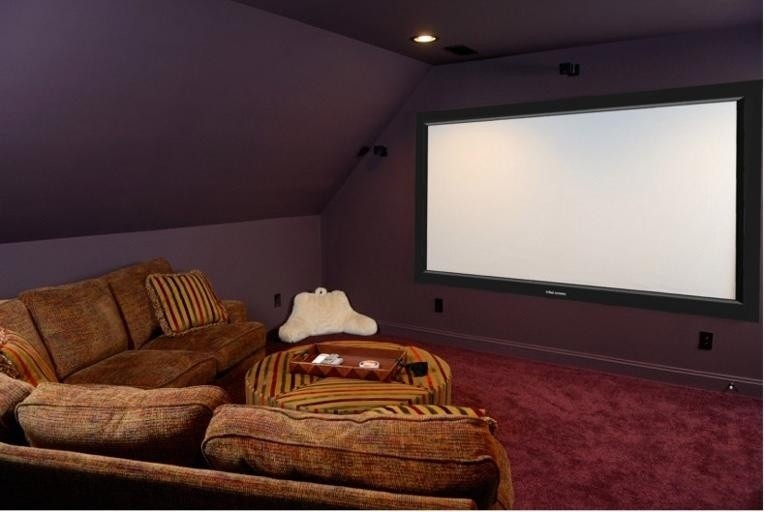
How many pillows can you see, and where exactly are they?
[0,326,61,387]
[145,269,229,337]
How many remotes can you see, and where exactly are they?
[320,351,339,366]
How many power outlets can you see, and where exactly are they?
[699,331,713,349]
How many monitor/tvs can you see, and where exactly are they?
[413,79,762,322]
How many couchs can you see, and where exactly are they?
[0,259,268,405]
[2,374,514,511]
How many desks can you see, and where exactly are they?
[245,342,452,413]
